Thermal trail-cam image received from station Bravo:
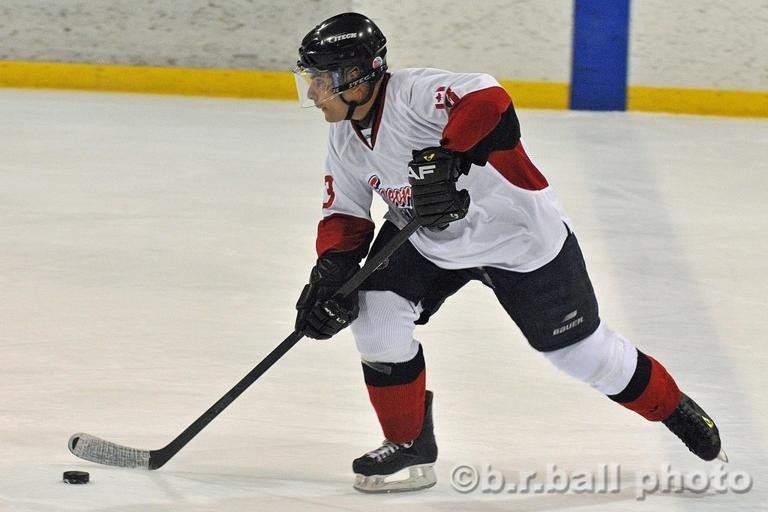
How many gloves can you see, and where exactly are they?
[295,251,361,340]
[407,146,471,230]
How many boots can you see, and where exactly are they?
[352,389,438,478]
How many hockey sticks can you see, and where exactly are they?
[68,217,420,469]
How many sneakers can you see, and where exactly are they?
[664,390,721,463]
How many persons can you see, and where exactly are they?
[293,12,723,476]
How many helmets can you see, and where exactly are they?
[293,11,387,110]
